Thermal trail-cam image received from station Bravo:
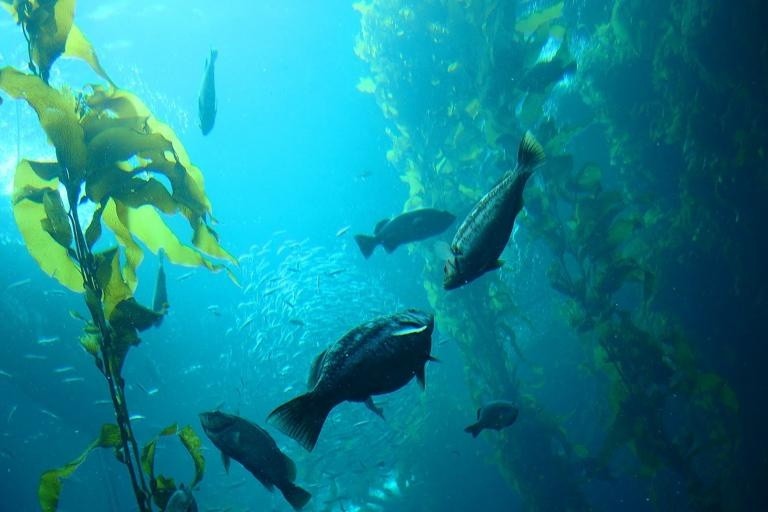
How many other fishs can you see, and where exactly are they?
[199,225,445,512]
